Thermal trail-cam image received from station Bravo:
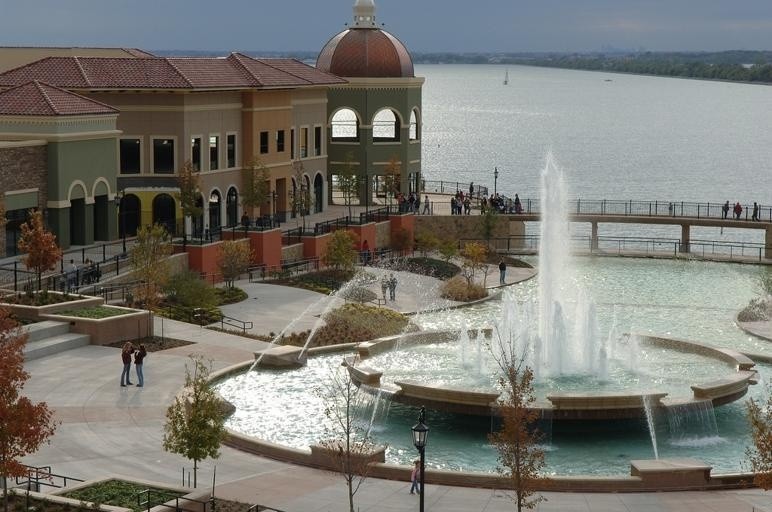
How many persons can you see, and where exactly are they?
[499,257,506,285]
[398,192,430,215]
[389,273,398,301]
[751,202,761,222]
[724,200,729,219]
[450,182,522,216]
[119,342,134,386]
[134,344,147,386]
[735,202,742,220]
[240,212,250,232]
[23,258,96,293]
[363,240,369,262]
[381,274,390,299]
[409,459,420,496]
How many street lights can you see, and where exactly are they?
[410,405,430,512]
[493,167,499,196]
